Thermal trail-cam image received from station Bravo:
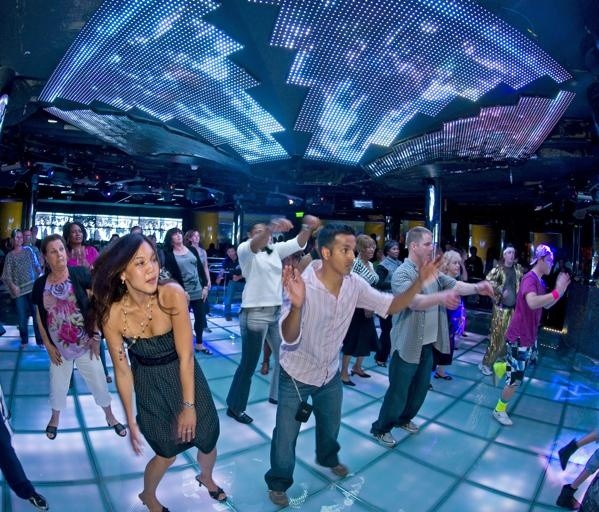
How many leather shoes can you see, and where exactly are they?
[269,397,279,403]
[435,371,452,380]
[227,407,253,424]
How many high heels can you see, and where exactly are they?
[340,376,356,386]
[138,491,169,512]
[374,356,386,367]
[195,346,212,355]
[260,360,269,374]
[196,473,227,502]
[350,368,371,378]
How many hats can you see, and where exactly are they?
[530,244,552,265]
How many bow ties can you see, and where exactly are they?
[261,246,274,254]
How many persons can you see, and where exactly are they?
[222,215,323,424]
[93,233,227,511]
[555,426,599,511]
[435,242,571,425]
[131,226,212,352]
[4,221,127,440]
[0,390,50,511]
[264,223,445,505]
[341,235,402,385]
[371,226,494,447]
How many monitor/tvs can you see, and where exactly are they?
[352,198,374,209]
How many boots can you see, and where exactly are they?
[556,484,581,510]
[558,439,577,470]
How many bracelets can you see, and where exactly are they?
[183,401,195,406]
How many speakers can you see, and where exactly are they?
[265,192,303,208]
[185,183,225,205]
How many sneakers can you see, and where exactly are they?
[479,362,493,376]
[372,426,397,447]
[319,459,348,476]
[493,409,513,426]
[269,489,289,506]
[30,493,49,510]
[400,421,420,432]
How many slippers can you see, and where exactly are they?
[46,423,58,440]
[107,420,128,436]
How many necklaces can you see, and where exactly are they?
[118,294,153,360]
